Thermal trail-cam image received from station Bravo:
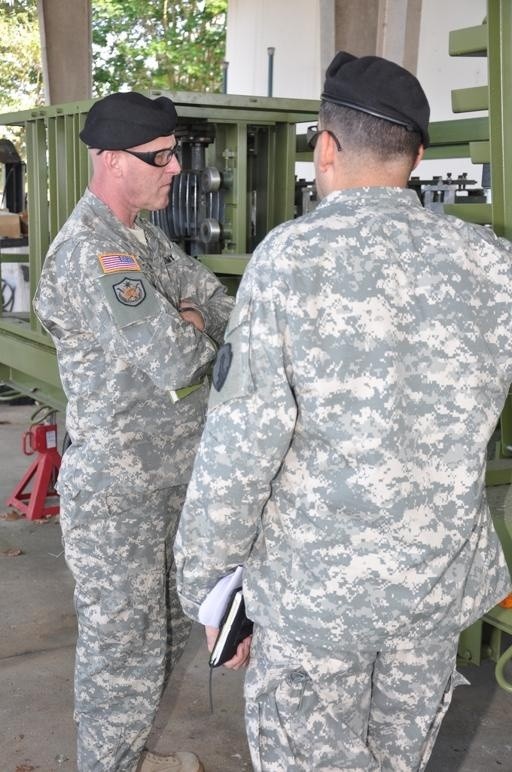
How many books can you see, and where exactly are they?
[208,586,254,668]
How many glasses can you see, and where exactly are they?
[98,147,181,168]
[306,126,342,151]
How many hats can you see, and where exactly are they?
[320,50,430,151]
[80,92,179,151]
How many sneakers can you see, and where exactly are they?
[134,743,205,772]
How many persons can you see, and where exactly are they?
[172,46,512,772]
[31,91,235,772]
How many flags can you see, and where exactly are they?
[99,254,141,274]
